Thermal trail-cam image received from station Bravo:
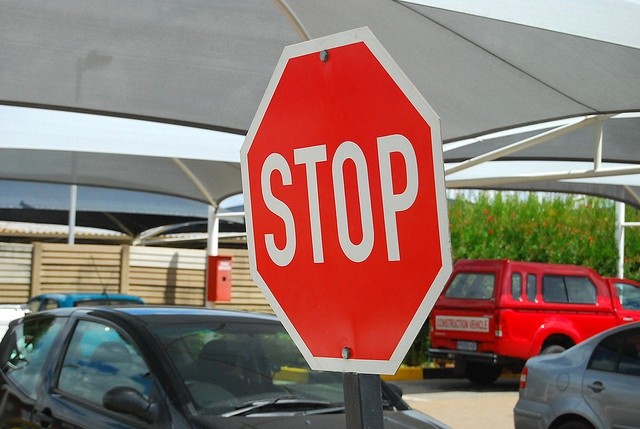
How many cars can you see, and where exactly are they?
[22,292,148,312]
[0,304,456,429]
[511,321,640,428]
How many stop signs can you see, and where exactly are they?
[239,26,455,376]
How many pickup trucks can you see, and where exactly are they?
[429,257,639,383]
